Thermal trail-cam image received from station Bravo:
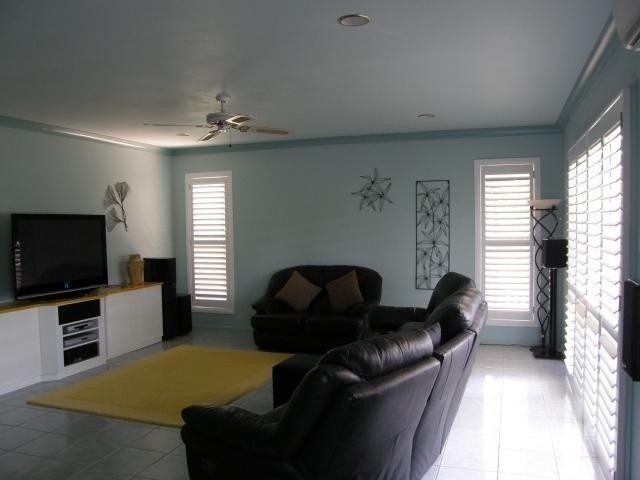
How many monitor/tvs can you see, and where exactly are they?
[10,212,108,301]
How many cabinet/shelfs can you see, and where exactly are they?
[0,282,165,396]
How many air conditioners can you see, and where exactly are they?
[613,0,640,53]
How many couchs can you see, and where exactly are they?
[180,271,488,479]
[249,264,384,350]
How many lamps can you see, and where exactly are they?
[527,199,568,360]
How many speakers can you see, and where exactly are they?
[143,257,177,341]
[621,278,640,382]
[176,294,193,336]
[540,238,568,270]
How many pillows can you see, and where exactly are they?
[273,270,321,314]
[323,270,366,304]
[328,292,357,315]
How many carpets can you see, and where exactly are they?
[26,344,296,428]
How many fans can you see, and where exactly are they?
[143,95,288,142]
